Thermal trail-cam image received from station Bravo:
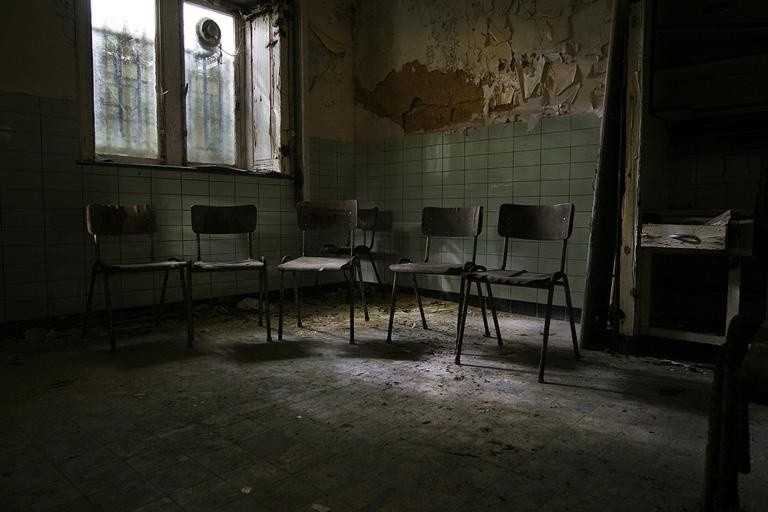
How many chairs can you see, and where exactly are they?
[386,206,491,344]
[308,208,385,322]
[277,200,359,344]
[80,203,188,351]
[454,204,581,384]
[170,205,272,345]
